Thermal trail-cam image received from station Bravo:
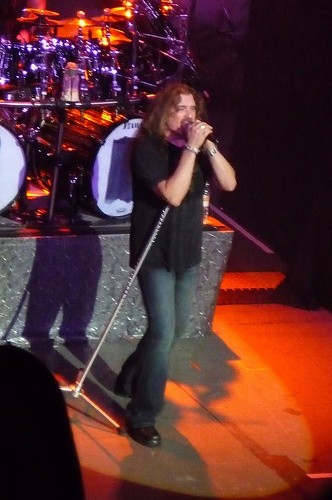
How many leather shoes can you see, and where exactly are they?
[114,380,132,397]
[129,424,160,447]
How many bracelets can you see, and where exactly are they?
[206,147,217,156]
[184,144,200,155]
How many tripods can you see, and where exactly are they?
[59,203,170,436]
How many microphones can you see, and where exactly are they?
[184,121,220,145]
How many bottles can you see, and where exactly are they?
[203,182,210,225]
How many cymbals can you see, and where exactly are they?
[91,14,125,23]
[62,18,102,27]
[17,7,65,27]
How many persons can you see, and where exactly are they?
[4,0,52,43]
[113,84,236,446]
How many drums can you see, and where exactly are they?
[0,37,116,103]
[24,103,144,219]
[0,106,28,215]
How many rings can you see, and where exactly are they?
[200,125,205,129]
